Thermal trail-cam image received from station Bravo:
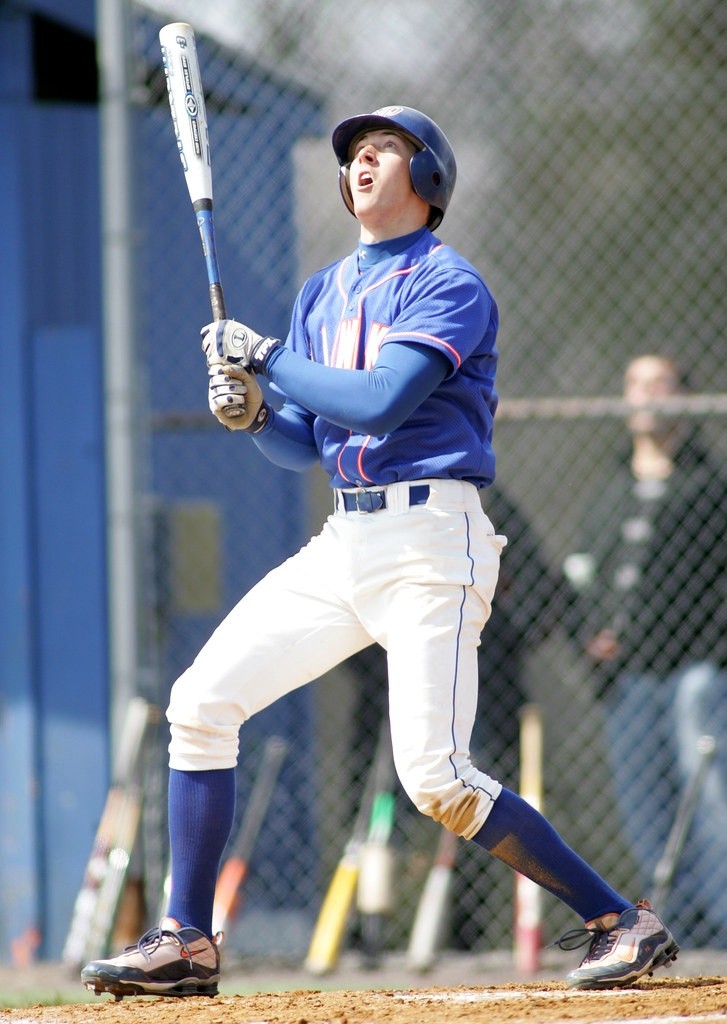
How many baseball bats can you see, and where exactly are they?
[407,765,482,972]
[161,19,227,324]
[212,736,290,951]
[650,739,718,919]
[62,697,168,973]
[512,706,542,971]
[304,718,399,976]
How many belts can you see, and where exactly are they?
[340,485,429,513]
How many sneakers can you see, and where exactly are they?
[543,899,681,988]
[81,917,223,1002]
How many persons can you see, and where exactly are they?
[77,105,679,997]
[339,475,620,983]
[588,356,726,975]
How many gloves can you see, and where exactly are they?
[208,361,268,434]
[200,320,284,376]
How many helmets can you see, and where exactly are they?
[332,104,457,233]
